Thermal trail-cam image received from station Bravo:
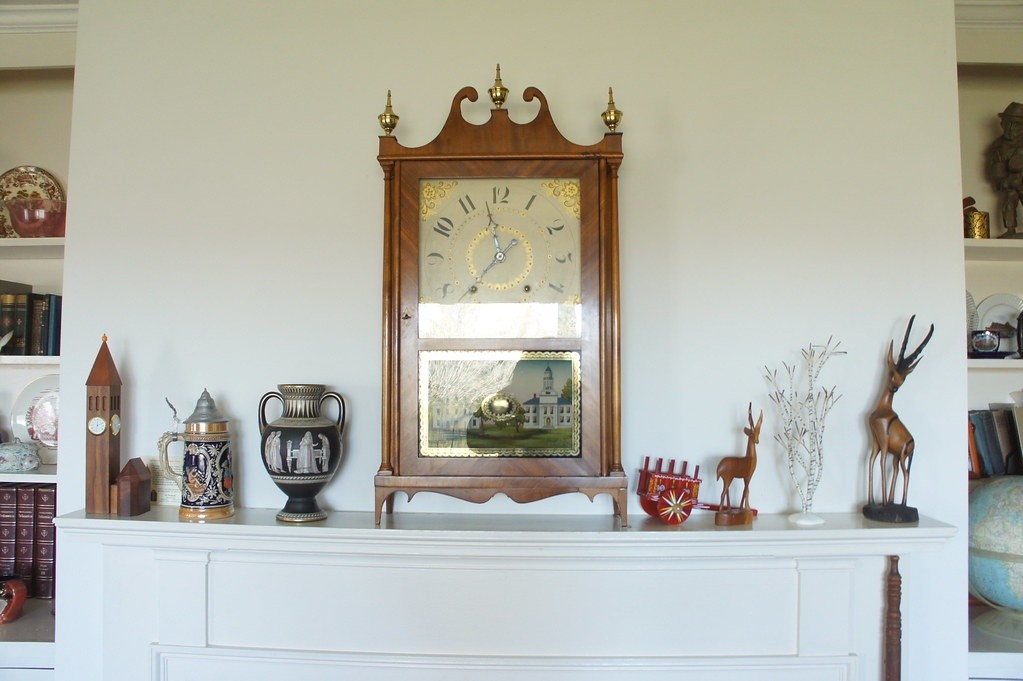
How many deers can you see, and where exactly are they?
[717,403,763,512]
[868,315,942,506]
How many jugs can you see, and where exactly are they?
[157,389,235,521]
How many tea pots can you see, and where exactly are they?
[0,437,42,473]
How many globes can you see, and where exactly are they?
[969,476,1023,648]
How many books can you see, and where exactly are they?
[0,293,62,356]
[967,403,1023,477]
[0,484,56,600]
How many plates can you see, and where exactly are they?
[9,373,60,466]
[968,349,1016,359]
[0,166,63,238]
[973,292,1023,359]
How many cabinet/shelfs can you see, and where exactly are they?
[0,237,68,668]
[964,235,1023,680]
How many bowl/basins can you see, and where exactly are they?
[4,198,66,238]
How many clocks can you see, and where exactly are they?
[372,65,629,529]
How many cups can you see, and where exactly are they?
[971,330,1000,352]
[965,210,990,238]
